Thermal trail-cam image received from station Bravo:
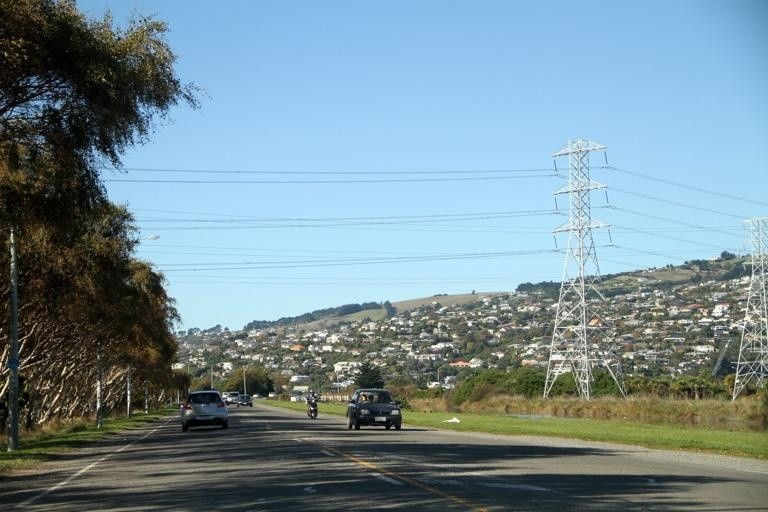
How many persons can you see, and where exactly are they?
[361,394,367,400]
[306,389,318,406]
[367,395,374,403]
[378,393,386,402]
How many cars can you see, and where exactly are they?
[347,388,401,430]
[181,391,229,432]
[237,394,252,407]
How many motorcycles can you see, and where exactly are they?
[307,397,321,419]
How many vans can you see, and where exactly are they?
[222,391,240,405]
